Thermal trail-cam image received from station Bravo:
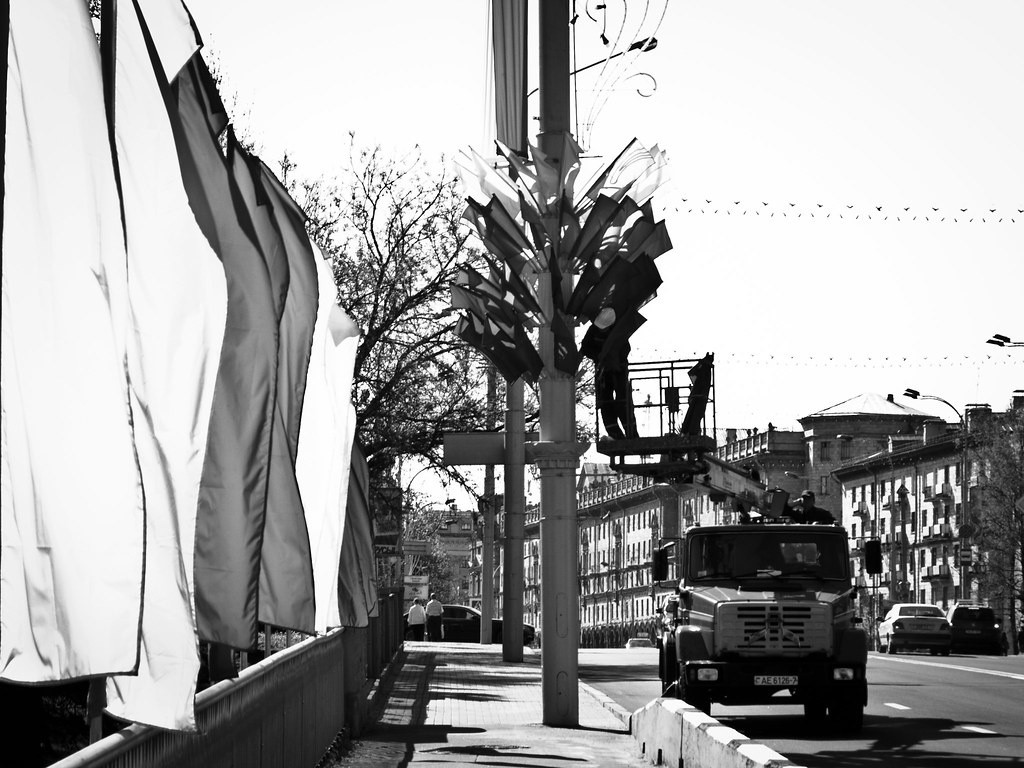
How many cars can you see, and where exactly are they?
[943,603,1004,655]
[403,603,535,649]
[873,600,952,658]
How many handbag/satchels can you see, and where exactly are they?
[441,624,444,639]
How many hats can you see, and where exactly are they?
[801,490,814,497]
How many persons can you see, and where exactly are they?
[784,490,836,524]
[580,307,641,438]
[426,593,444,642]
[407,598,426,641]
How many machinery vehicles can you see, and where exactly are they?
[596,357,884,733]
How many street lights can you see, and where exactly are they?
[903,387,969,597]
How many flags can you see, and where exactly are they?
[680,353,714,435]
[0,1,380,732]
[447,139,674,388]
